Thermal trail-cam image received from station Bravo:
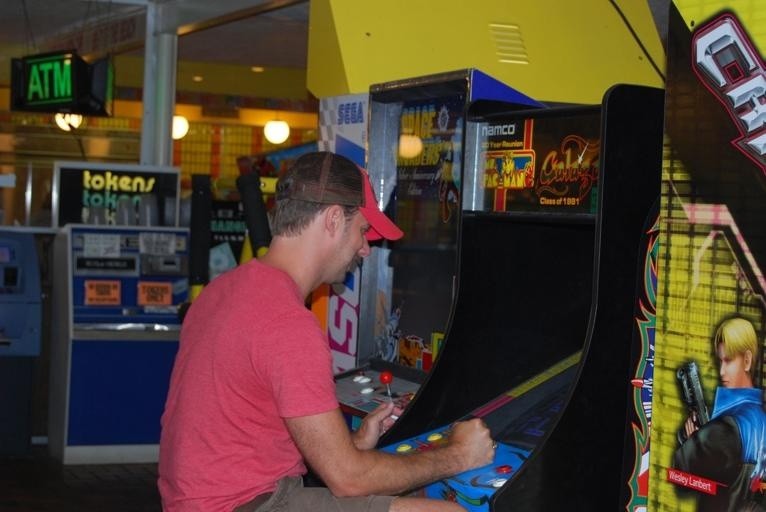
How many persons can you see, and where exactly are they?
[157,150,495,511]
[667,318,765,511]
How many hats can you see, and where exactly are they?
[276,152,405,241]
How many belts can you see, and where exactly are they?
[234,492,273,512]
